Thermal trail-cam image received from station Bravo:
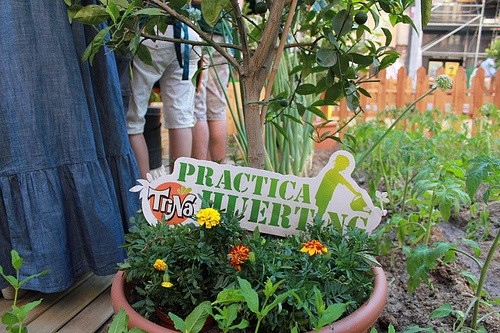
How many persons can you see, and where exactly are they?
[191,3,233,164]
[125,0,205,181]
[0,0,148,301]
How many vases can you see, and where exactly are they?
[111,259,388,333]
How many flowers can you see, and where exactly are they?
[114,210,382,333]
